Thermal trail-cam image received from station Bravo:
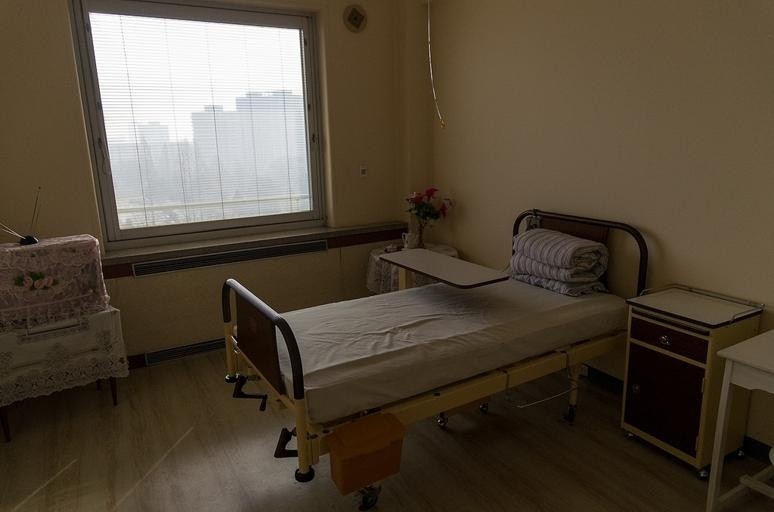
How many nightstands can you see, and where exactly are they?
[369,243,458,288]
[620,288,765,465]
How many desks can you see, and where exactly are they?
[1,304,118,449]
[709,333,774,511]
[380,249,511,291]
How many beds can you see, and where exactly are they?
[217,210,650,506]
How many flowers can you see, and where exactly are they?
[405,186,454,224]
